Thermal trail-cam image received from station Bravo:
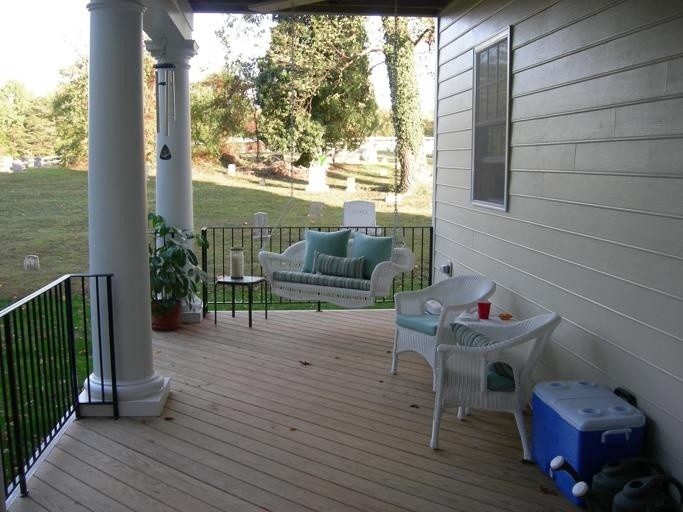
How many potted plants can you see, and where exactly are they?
[147,212,209,331]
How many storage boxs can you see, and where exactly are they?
[531,380,646,507]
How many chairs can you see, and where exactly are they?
[394,274,497,393]
[429,313,562,460]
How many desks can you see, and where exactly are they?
[214,276,268,327]
[454,313,521,416]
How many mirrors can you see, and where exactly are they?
[469,26,511,212]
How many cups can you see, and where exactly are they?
[477,300,492,320]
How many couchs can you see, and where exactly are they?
[259,239,414,309]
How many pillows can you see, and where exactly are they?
[306,230,392,279]
[450,323,514,380]
[487,371,515,391]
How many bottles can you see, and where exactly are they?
[230,246,244,278]
